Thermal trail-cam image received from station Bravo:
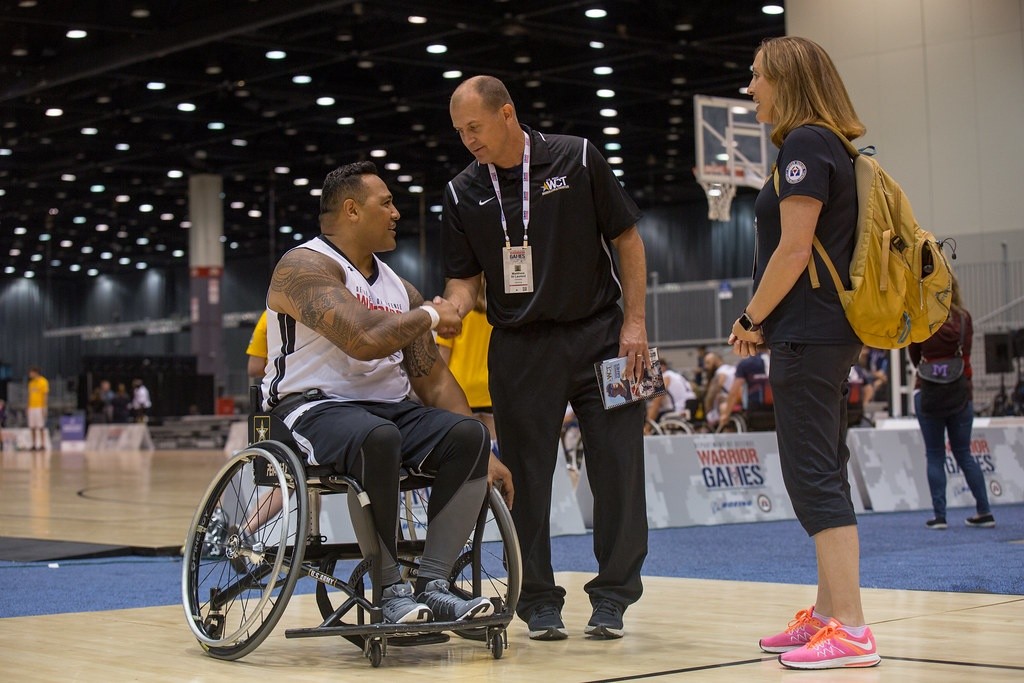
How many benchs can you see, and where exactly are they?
[150,417,232,450]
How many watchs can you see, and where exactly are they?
[739,308,760,331]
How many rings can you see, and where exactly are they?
[636,354,643,357]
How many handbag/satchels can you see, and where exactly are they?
[916,360,969,418]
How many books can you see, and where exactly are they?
[594,347,666,410]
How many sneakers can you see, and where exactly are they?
[759,607,828,653]
[527,601,568,640]
[584,597,626,638]
[414,579,495,622]
[777,618,881,669]
[925,518,948,529]
[382,579,435,636]
[964,514,995,528]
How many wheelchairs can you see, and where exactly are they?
[179,388,523,667]
[561,411,747,473]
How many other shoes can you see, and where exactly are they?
[228,524,252,575]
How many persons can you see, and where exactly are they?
[608,359,664,401]
[728,34,881,668]
[65,378,153,425]
[229,308,321,573]
[848,344,892,427]
[27,367,49,451]
[644,343,776,436]
[433,74,650,640]
[908,276,995,529]
[434,269,498,447]
[260,160,513,634]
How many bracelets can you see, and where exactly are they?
[420,305,439,330]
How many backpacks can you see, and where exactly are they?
[774,120,952,350]
